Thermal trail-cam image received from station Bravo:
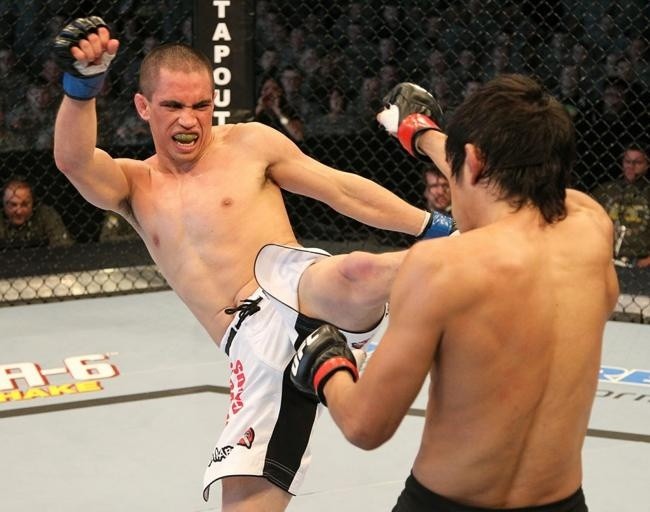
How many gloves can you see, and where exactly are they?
[50,13,123,100]
[374,78,446,161]
[288,320,359,407]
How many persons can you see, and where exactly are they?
[0,0,191,251]
[255,1,650,282]
[281,71,626,509]
[51,11,465,512]
